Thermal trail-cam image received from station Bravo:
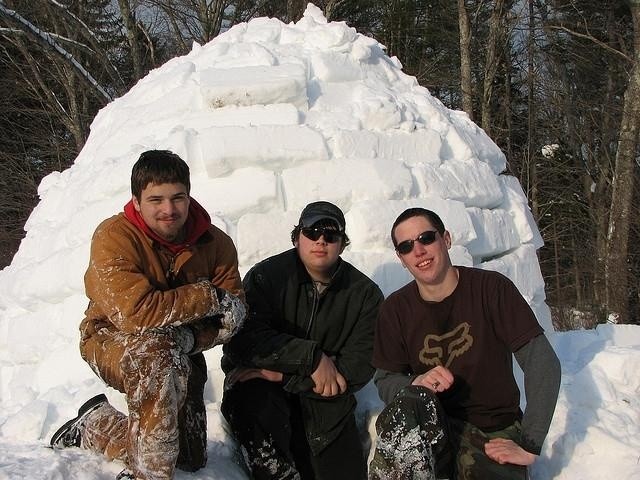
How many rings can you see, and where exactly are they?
[432,382,440,389]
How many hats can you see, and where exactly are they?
[298,200,345,229]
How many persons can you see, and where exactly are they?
[50,150,250,480]
[369,208,561,480]
[221,201,384,480]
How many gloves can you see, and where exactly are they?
[149,324,198,354]
[213,287,250,346]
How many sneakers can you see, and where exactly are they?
[116,466,135,479]
[49,393,109,451]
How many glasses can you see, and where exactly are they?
[300,228,346,243]
[395,229,443,254]
